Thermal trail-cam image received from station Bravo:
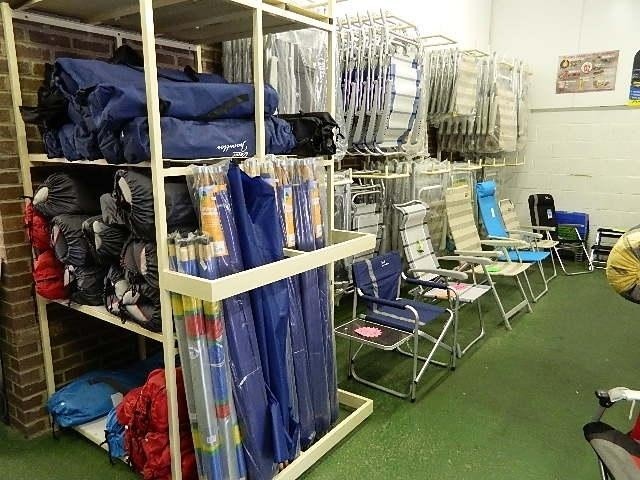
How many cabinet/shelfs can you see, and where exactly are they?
[0,0,375,480]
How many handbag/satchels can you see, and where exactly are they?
[23,169,199,330]
[276,111,345,160]
[37,58,295,163]
[105,366,196,480]
[47,354,182,427]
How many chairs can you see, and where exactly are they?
[445,182,533,330]
[582,386,640,480]
[553,209,589,267]
[528,192,594,278]
[474,180,548,306]
[496,195,557,288]
[393,201,493,360]
[333,256,457,396]
[261,160,507,287]
[225,10,531,157]
